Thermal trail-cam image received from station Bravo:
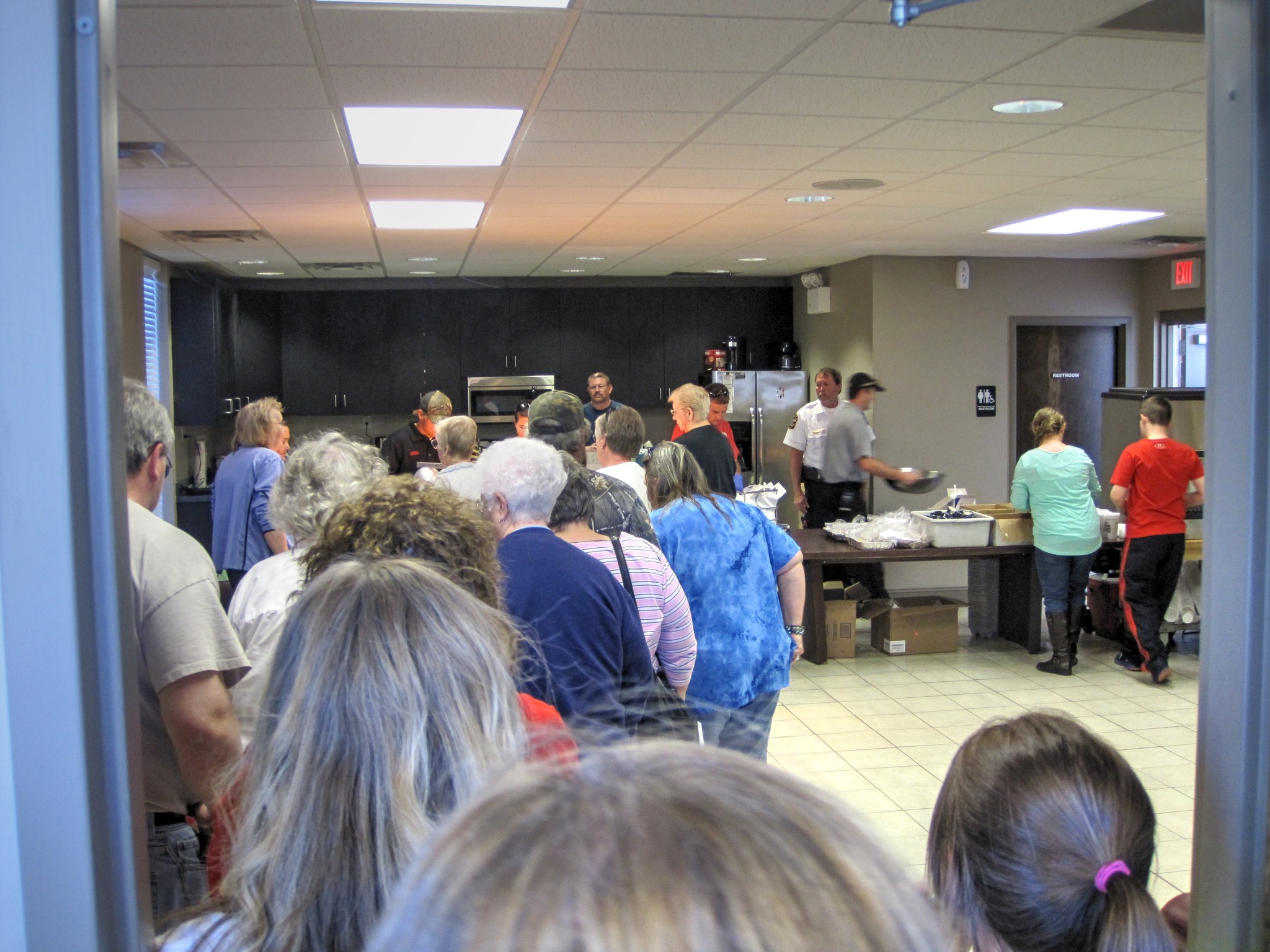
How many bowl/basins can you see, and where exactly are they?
[924,506,976,519]
[887,467,947,494]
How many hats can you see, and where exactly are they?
[851,373,884,392]
[527,390,585,435]
[420,391,453,424]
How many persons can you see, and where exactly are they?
[381,371,807,764]
[154,555,535,952]
[205,475,580,910]
[120,375,253,934]
[363,734,961,952]
[227,429,389,755]
[1109,396,1205,684]
[1010,408,1102,676]
[782,366,923,587]
[211,396,295,613]
[926,706,1191,952]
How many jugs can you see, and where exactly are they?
[781,355,801,368]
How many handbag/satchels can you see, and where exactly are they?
[638,674,700,746]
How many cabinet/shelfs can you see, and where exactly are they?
[170,277,793,426]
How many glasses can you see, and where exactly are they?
[274,419,285,428]
[670,407,688,416]
[516,402,531,412]
[605,405,618,423]
[147,446,173,478]
[588,384,609,391]
[709,388,730,397]
[476,496,497,514]
[431,439,440,449]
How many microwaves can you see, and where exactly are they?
[467,375,554,423]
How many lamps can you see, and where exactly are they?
[802,273,830,314]
[1171,258,1201,290]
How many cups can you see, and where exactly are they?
[1096,508,1126,540]
[193,441,207,487]
[1164,560,1200,623]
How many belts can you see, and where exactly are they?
[147,810,188,827]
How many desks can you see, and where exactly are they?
[790,528,1124,665]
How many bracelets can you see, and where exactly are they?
[784,626,805,635]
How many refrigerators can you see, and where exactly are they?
[706,370,809,529]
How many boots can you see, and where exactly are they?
[1036,610,1073,677]
[1066,604,1087,666]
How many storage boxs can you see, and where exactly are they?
[1087,574,1126,642]
[823,581,872,658]
[910,502,1033,548]
[857,595,972,656]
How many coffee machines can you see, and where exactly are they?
[780,342,802,371]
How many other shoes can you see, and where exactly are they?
[1152,665,1171,682]
[1114,653,1141,671]
